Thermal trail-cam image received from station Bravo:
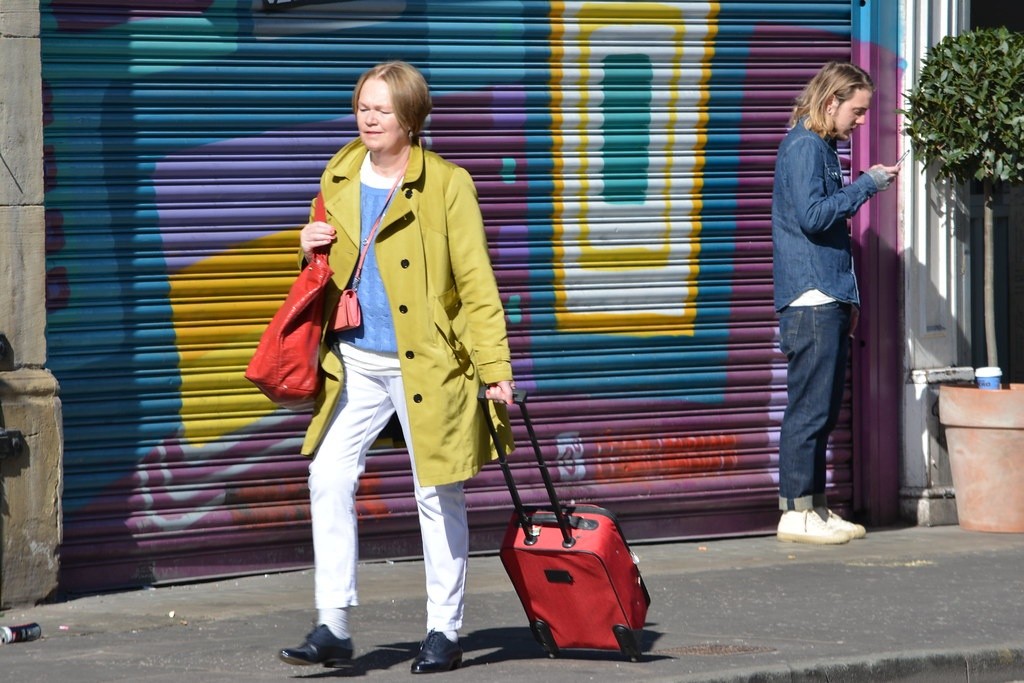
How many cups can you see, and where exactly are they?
[975,366,1003,391]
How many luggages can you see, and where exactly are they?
[476,386,651,662]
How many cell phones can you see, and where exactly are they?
[895,150,910,166]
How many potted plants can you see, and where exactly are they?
[898,25,1024,534]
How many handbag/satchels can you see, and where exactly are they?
[244,191,334,412]
[327,289,361,333]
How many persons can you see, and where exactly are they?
[279,61,516,674]
[775,61,900,545]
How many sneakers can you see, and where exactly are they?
[813,505,865,539]
[777,509,849,544]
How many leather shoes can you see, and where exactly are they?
[278,624,353,666]
[411,630,463,672]
